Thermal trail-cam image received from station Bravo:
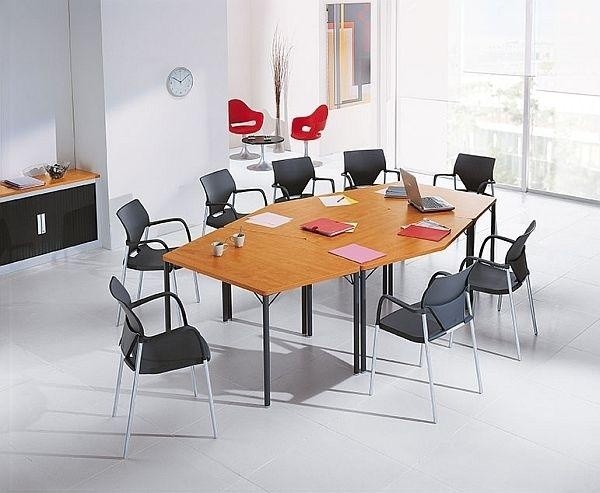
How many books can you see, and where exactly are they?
[299,216,355,237]
[383,182,410,198]
[3,171,48,190]
[396,223,450,243]
[412,217,452,233]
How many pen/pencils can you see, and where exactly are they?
[422,217,452,230]
[336,197,345,203]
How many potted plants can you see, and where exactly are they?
[269,19,293,153]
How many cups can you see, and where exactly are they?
[229,232,245,247]
[211,241,228,256]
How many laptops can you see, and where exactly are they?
[400,168,455,213]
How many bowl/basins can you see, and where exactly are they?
[43,160,71,179]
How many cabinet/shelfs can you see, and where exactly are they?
[0,167,102,275]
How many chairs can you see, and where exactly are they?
[369,258,482,424]
[290,103,328,167]
[199,168,268,237]
[271,157,335,204]
[458,219,538,361]
[433,153,496,197]
[109,276,218,459]
[228,97,265,160]
[341,149,400,191]
[115,198,200,327]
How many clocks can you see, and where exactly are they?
[166,64,193,96]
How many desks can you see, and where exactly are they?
[162,180,497,407]
[241,135,284,171]
